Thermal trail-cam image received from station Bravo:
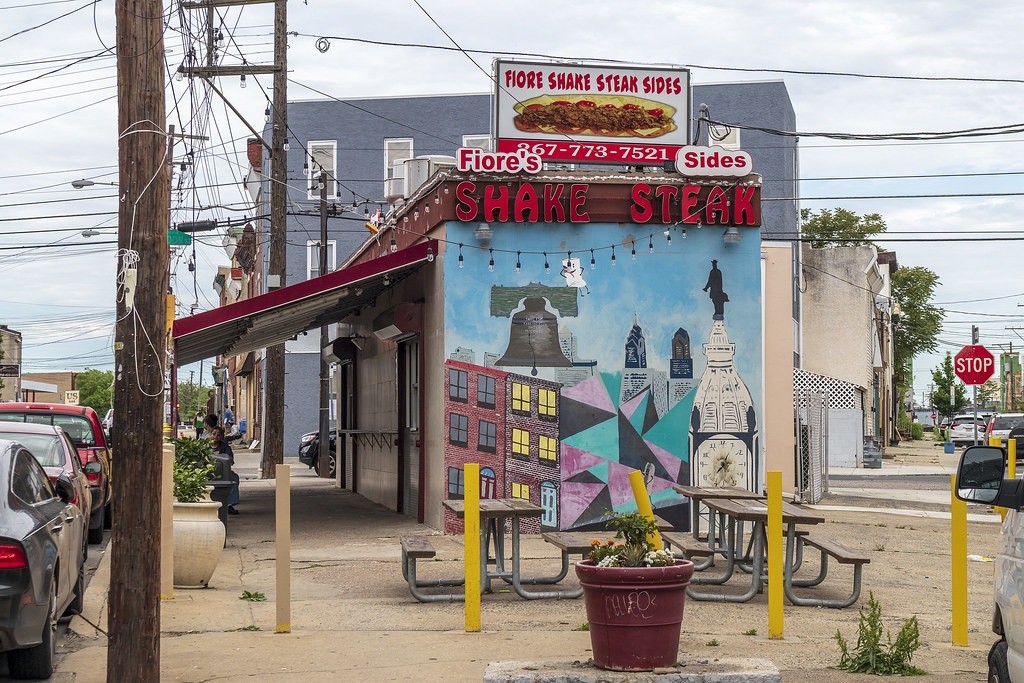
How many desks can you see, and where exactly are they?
[442,497,546,599]
[702,498,825,606]
[673,485,767,575]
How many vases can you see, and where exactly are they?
[574,558,694,671]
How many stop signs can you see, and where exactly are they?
[954,344,995,386]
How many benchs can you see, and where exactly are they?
[542,532,606,598]
[399,534,436,602]
[801,534,871,608]
[654,515,675,532]
[764,521,809,571]
[661,529,715,600]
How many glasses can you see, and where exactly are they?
[213,433,220,436]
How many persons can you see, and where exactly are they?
[209,426,239,515]
[224,404,235,434]
[193,408,218,439]
[224,418,242,444]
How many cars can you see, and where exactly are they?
[102,408,114,444]
[948,414,987,444]
[939,417,952,436]
[2,402,111,543]
[952,447,1024,683]
[0,422,101,536]
[1,443,90,680]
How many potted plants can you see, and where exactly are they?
[164,435,227,590]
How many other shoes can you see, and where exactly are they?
[228,505,238,515]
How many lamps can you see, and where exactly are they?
[475,222,494,240]
[351,338,365,350]
[723,226,742,243]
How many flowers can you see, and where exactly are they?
[589,507,675,567]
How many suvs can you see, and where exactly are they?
[977,413,1024,463]
